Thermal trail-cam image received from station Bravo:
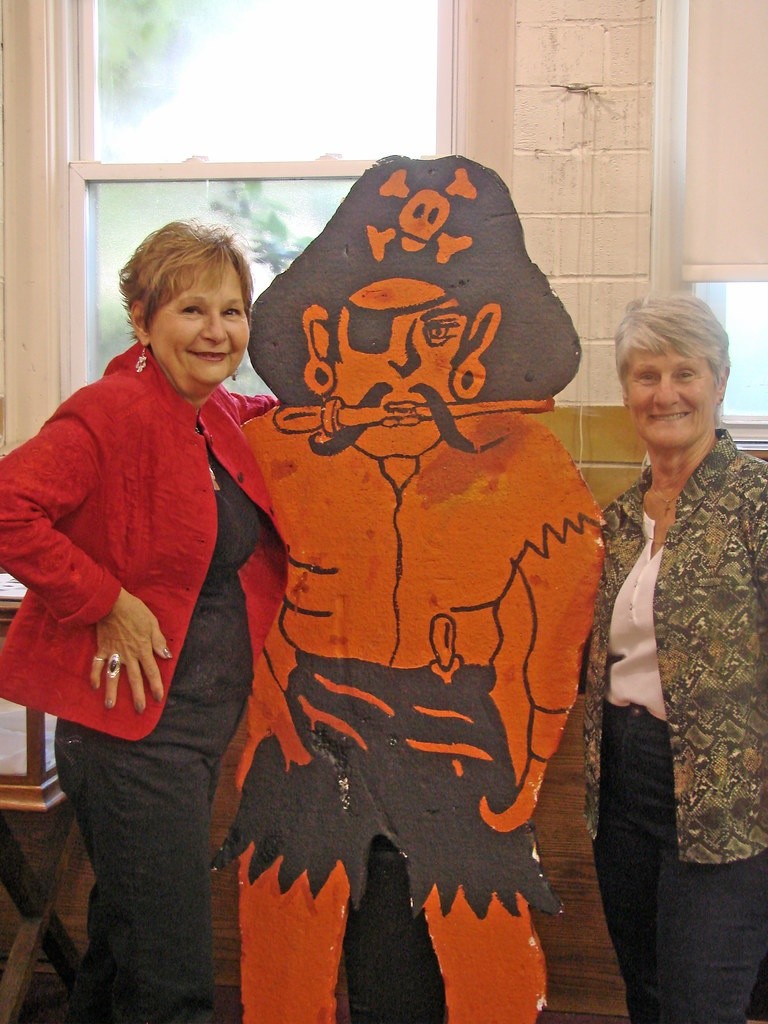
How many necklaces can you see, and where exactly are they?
[650,486,682,515]
[640,490,666,545]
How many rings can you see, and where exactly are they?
[106,652,121,678]
[93,656,104,661]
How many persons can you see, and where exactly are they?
[1,218,290,1024]
[579,293,767,1024]
